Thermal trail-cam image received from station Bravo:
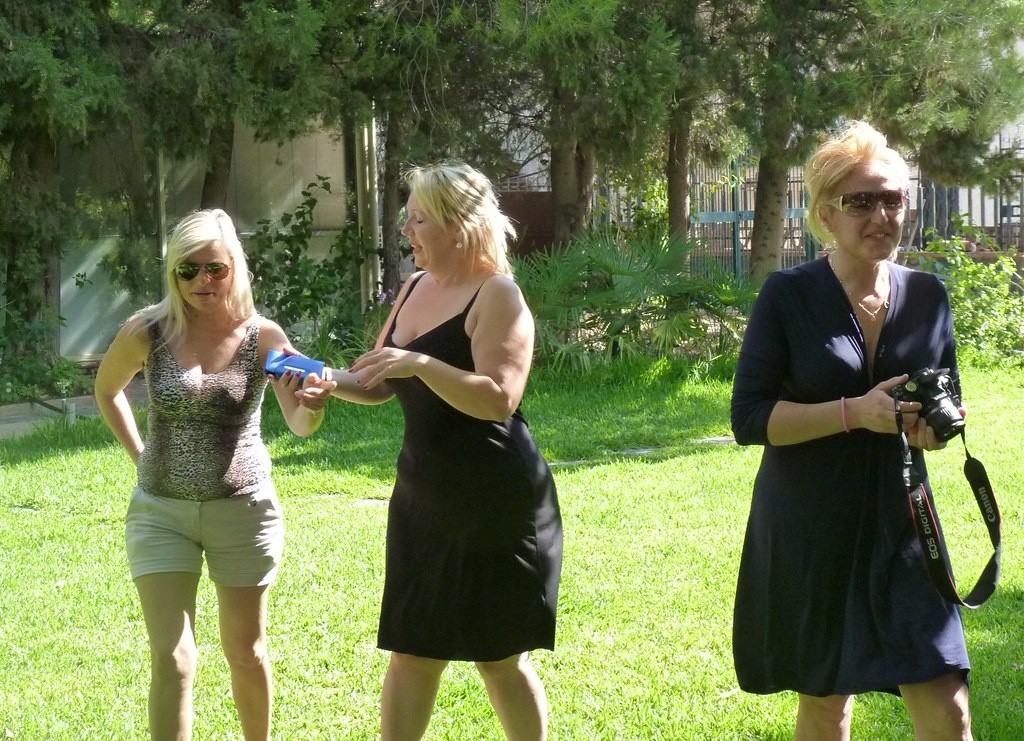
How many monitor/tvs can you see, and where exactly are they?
[891,366,968,441]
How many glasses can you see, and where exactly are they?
[825,189,907,216]
[175,262,232,281]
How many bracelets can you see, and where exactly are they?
[130,445,142,456]
[841,397,849,432]
[325,372,327,381]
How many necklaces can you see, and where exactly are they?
[185,339,217,357]
[828,257,887,321]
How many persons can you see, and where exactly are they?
[268,160,564,741]
[729,119,972,741]
[93,208,337,741]
